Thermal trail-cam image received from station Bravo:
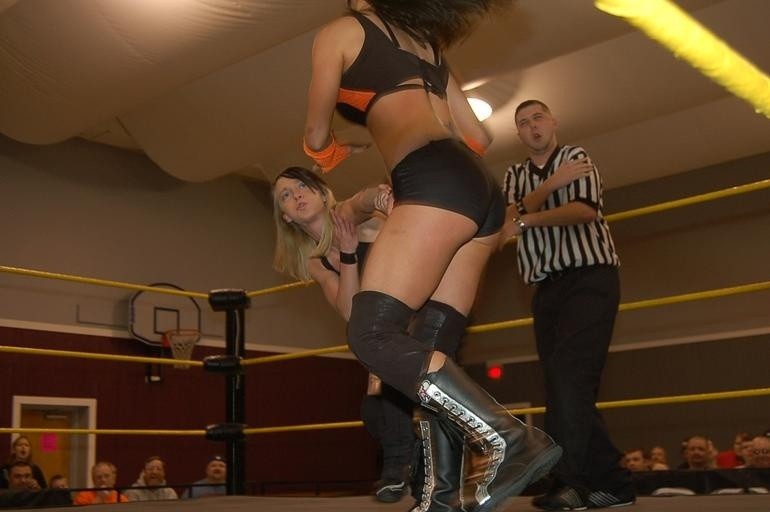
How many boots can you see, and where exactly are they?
[375,356,563,511]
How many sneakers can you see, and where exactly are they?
[517,467,637,512]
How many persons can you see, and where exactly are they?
[500,101,636,511]
[303,0,564,512]
[0,435,228,508]
[614,430,770,498]
[270,165,426,502]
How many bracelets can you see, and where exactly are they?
[339,250,358,265]
[515,198,527,216]
[513,216,527,233]
